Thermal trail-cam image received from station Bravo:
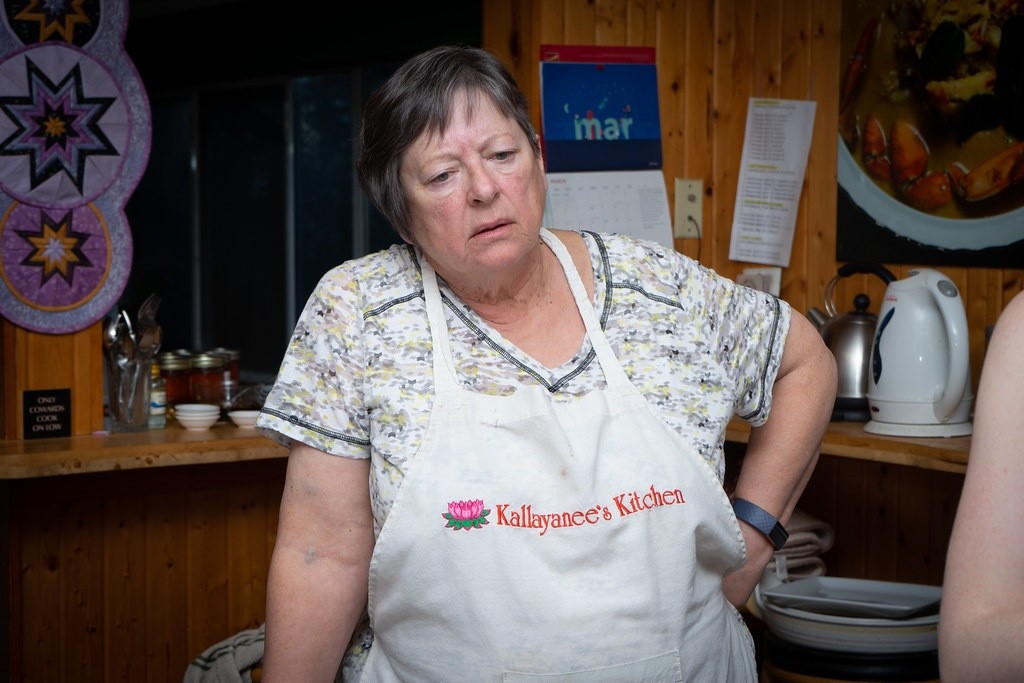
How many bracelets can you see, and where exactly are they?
[729,497,790,551]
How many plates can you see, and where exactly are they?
[761,600,939,654]
[837,130,1024,251]
[763,573,944,621]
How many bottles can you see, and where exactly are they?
[151,345,241,420]
[149,364,167,429]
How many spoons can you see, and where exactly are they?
[103,291,162,422]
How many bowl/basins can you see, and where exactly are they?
[175,404,220,430]
[227,410,260,430]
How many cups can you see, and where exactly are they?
[106,352,152,434]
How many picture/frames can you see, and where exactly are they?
[837,0,1024,268]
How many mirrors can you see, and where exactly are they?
[108,0,486,372]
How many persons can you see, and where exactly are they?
[937,290,1023,682]
[253,48,840,682]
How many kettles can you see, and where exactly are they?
[806,258,898,425]
[862,267,974,440]
